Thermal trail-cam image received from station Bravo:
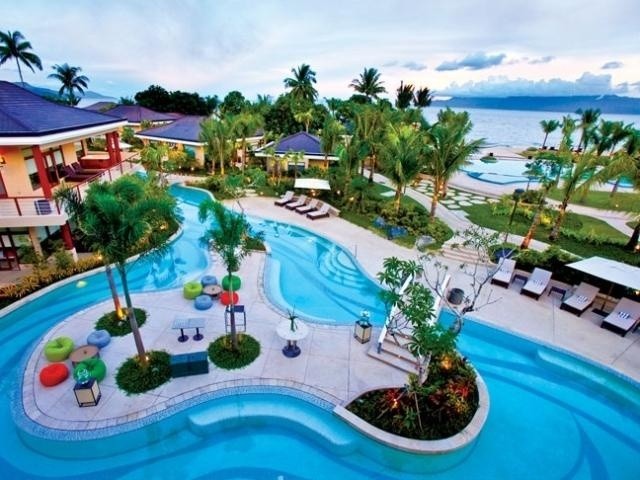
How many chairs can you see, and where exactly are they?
[274,191,331,221]
[560,282,600,317]
[71,162,99,174]
[491,257,516,289]
[600,297,640,337]
[63,165,94,177]
[520,267,552,300]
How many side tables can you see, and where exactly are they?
[548,286,566,301]
[512,274,528,286]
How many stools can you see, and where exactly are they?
[201,275,217,287]
[221,292,239,305]
[184,281,202,299]
[87,330,111,348]
[45,337,73,361]
[195,295,213,311]
[223,274,241,291]
[40,364,69,386]
[73,358,107,383]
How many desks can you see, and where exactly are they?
[276,320,308,358]
[172,318,205,343]
